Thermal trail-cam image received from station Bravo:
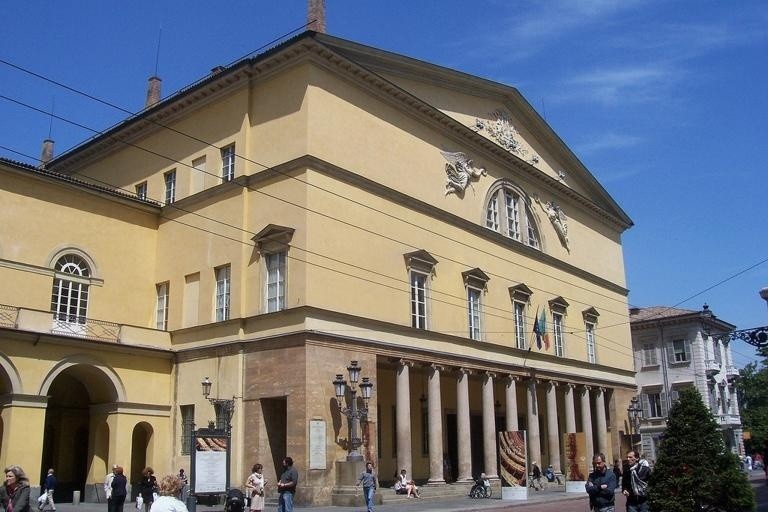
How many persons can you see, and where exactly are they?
[148,473,190,512]
[0,464,38,512]
[103,464,119,512]
[36,467,60,511]
[176,468,187,502]
[584,451,617,512]
[637,454,650,467]
[613,459,623,488]
[529,461,544,491]
[244,463,269,511]
[355,461,376,512]
[622,449,654,512]
[400,469,415,486]
[534,194,571,254]
[444,159,487,197]
[138,467,157,512]
[276,456,298,512]
[393,473,406,494]
[544,464,555,482]
[111,467,128,512]
[737,453,765,470]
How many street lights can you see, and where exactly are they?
[332,360,374,458]
[629,397,643,462]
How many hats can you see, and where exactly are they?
[112,465,118,470]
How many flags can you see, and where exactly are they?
[536,309,552,349]
[528,310,542,356]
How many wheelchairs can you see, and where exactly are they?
[468,479,492,499]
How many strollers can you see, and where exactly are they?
[224,488,245,512]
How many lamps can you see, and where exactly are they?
[201,376,243,425]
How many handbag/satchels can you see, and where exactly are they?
[135,493,143,510]
